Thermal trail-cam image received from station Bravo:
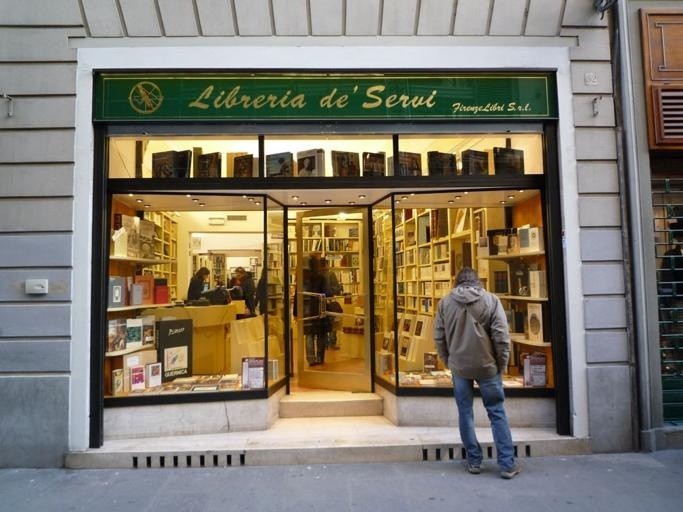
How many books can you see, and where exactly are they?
[267,205,366,329]
[104,205,257,403]
[147,144,527,178]
[374,205,553,390]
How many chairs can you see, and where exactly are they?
[250,242,297,310]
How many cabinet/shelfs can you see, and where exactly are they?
[372,207,507,368]
[225,313,286,375]
[102,211,170,356]
[296,219,361,303]
[475,223,550,347]
[196,254,228,290]
[138,210,178,299]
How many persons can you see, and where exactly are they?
[434,265,524,479]
[255,263,267,317]
[293,252,342,366]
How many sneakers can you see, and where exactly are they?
[468,463,481,473]
[500,458,524,478]
